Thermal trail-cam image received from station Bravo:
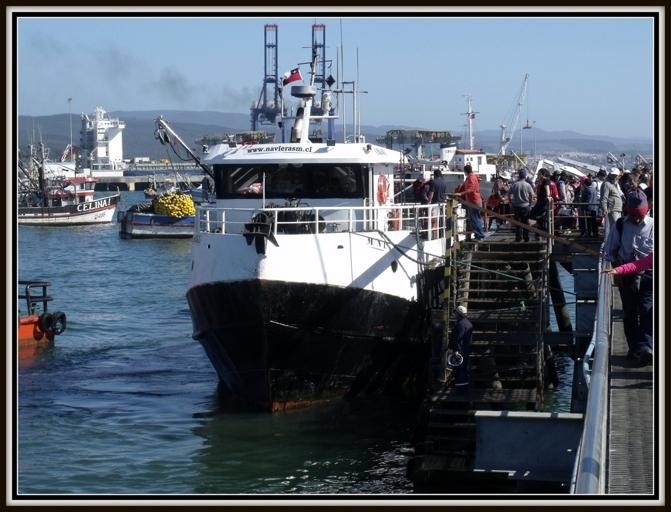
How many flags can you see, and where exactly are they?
[282,67,302,85]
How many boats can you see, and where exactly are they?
[19,281,65,357]
[121,172,204,236]
[18,142,120,225]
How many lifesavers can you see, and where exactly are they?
[379,175,388,205]
[38,312,54,334]
[52,311,67,334]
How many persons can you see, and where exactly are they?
[412,168,446,239]
[450,305,473,385]
[459,164,485,240]
[485,166,653,241]
[605,188,651,366]
[290,106,304,144]
[609,252,654,276]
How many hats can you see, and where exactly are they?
[499,171,511,179]
[519,169,527,177]
[610,167,621,175]
[455,305,467,317]
[626,188,649,217]
